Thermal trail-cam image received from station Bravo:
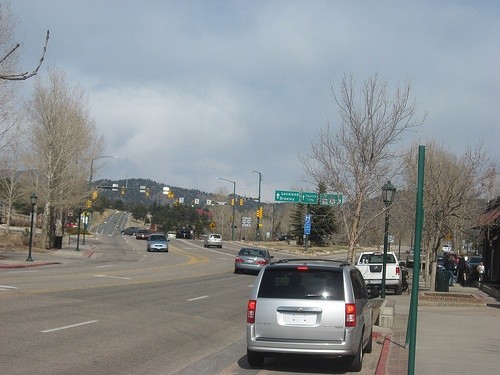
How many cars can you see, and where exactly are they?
[120,226,194,241]
[405,244,483,286]
[146,233,170,252]
[234,247,275,275]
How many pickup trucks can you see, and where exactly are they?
[354,250,406,295]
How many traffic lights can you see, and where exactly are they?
[86,200,92,208]
[191,201,195,208]
[230,216,233,223]
[92,191,98,200]
[256,206,263,219]
[176,201,179,208]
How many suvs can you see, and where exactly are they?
[245,258,380,372]
[203,234,222,249]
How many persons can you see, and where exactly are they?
[443,254,485,286]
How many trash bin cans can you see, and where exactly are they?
[435,272,452,292]
[55,236,62,249]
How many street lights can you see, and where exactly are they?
[75,207,84,252]
[380,180,397,298]
[218,178,236,242]
[266,200,275,238]
[82,155,118,245]
[251,170,262,242]
[26,192,38,262]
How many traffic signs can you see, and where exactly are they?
[274,189,300,203]
[300,191,319,204]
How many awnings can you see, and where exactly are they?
[471,205,500,229]
